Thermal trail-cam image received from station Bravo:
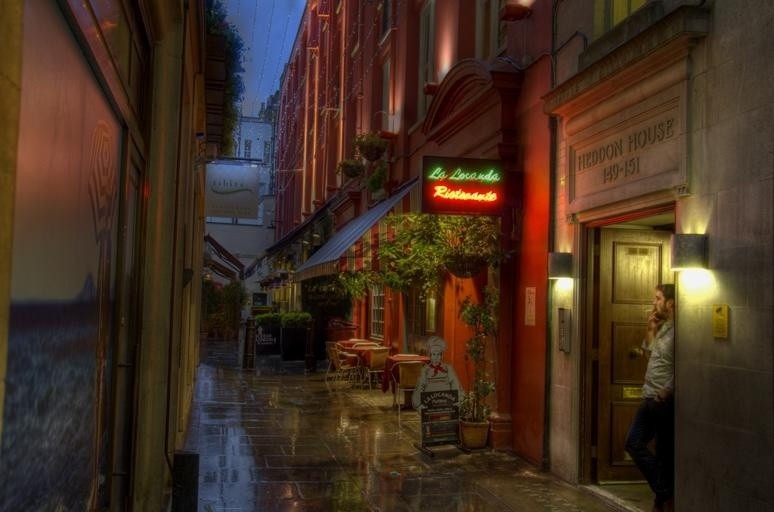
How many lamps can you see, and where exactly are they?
[670,233,710,271]
[546,252,574,279]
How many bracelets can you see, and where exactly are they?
[656,394,664,403]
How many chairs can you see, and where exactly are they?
[321,337,432,429]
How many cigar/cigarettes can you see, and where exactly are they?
[646,309,654,312]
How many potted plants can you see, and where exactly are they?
[340,132,387,178]
[427,215,519,279]
[254,311,312,360]
[458,294,503,448]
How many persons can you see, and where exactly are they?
[410,334,462,411]
[620,282,673,512]
